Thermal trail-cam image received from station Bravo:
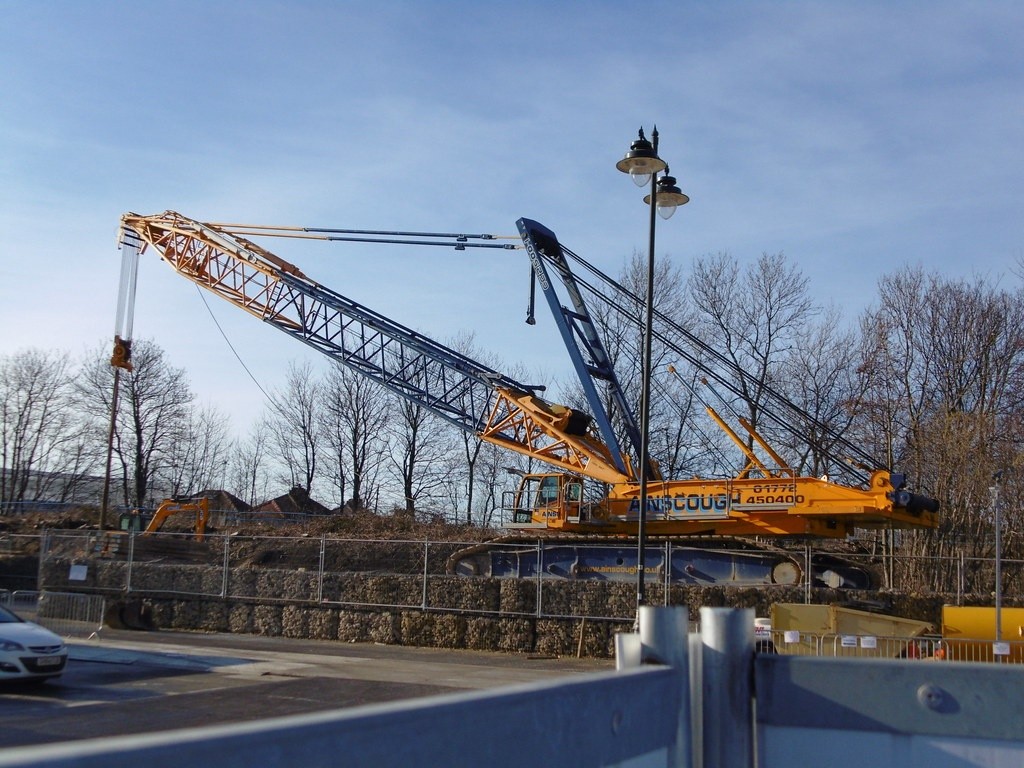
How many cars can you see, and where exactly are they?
[0,604,69,691]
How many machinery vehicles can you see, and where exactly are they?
[94,492,216,566]
[98,207,942,589]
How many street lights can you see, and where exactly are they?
[989,468,1005,662]
[614,124,690,636]
